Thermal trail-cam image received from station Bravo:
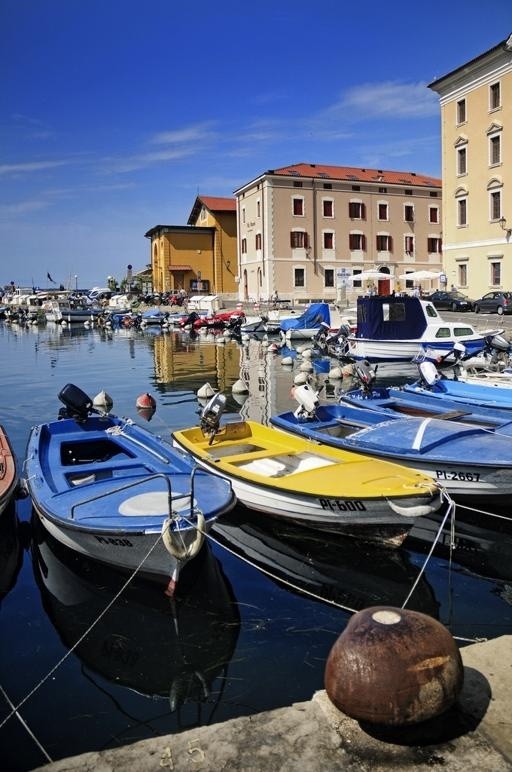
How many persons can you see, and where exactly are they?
[450,284,457,292]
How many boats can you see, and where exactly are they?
[280,295,504,359]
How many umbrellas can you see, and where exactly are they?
[348,268,394,298]
[399,269,443,296]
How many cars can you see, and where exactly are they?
[472,291,512,315]
[424,291,472,311]
